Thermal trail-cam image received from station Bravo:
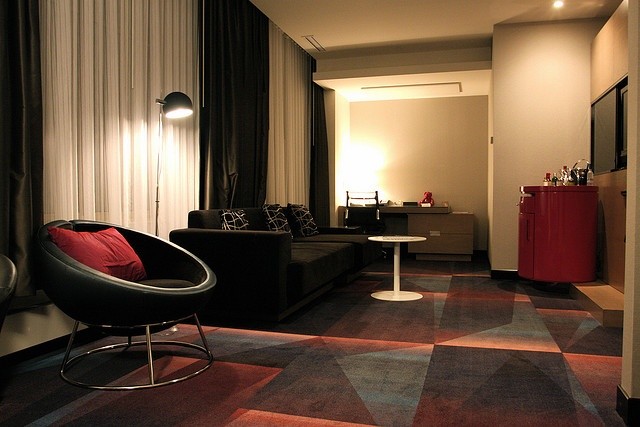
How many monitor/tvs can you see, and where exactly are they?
[590,87,623,175]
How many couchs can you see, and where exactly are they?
[169,206,378,325]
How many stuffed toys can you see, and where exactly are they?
[418,191,434,206]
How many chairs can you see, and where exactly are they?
[0,253,16,309]
[36,220,217,393]
[344,190,385,234]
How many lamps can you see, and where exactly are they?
[153,92,195,242]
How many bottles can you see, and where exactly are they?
[586,167,594,186]
[542,177,549,186]
[545,173,551,182]
[552,173,558,186]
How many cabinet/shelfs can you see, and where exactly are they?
[337,206,474,261]
[519,184,599,283]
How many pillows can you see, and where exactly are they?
[46,228,147,280]
[222,209,250,231]
[289,203,318,236]
[262,203,296,240]
